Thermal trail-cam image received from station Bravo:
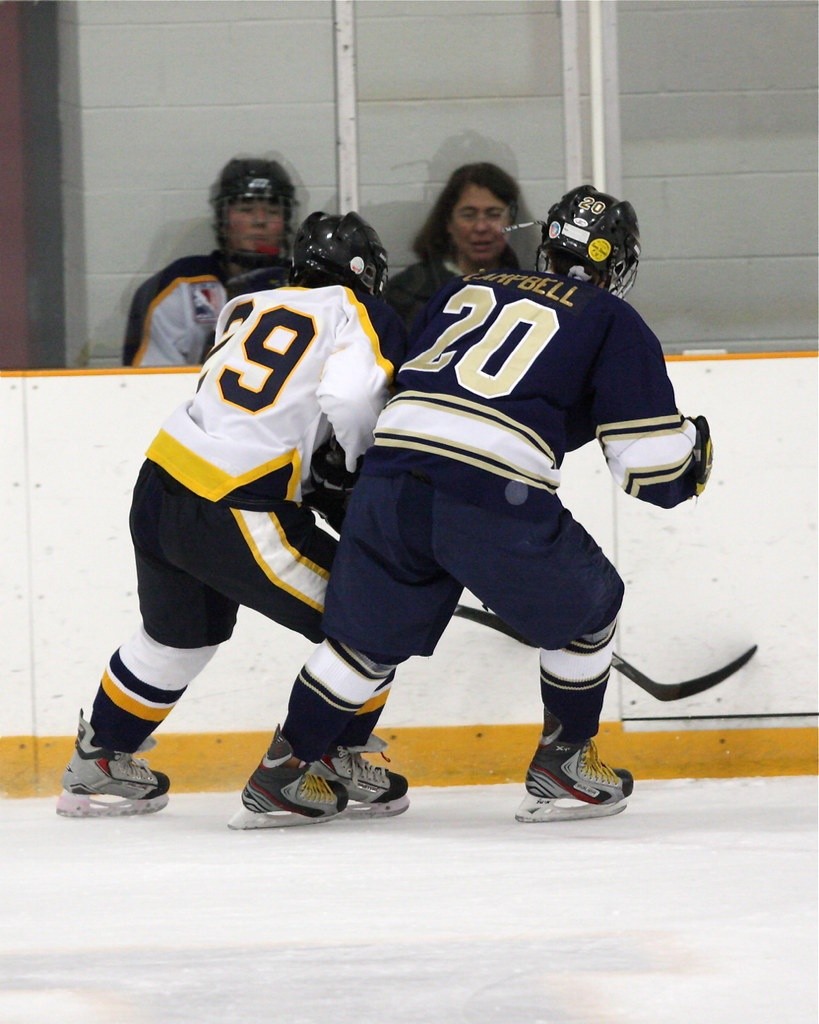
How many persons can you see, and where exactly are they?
[57,210,411,822]
[383,163,521,334]
[124,158,297,368]
[228,184,713,831]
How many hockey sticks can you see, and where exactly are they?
[612,640,758,703]
[450,604,539,647]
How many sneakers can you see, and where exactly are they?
[227,719,349,830]
[514,705,634,822]
[304,731,410,814]
[55,707,170,818]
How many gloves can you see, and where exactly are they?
[690,417,714,498]
[310,437,366,505]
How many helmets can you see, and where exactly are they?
[293,211,389,298]
[211,158,299,268]
[535,185,640,296]
